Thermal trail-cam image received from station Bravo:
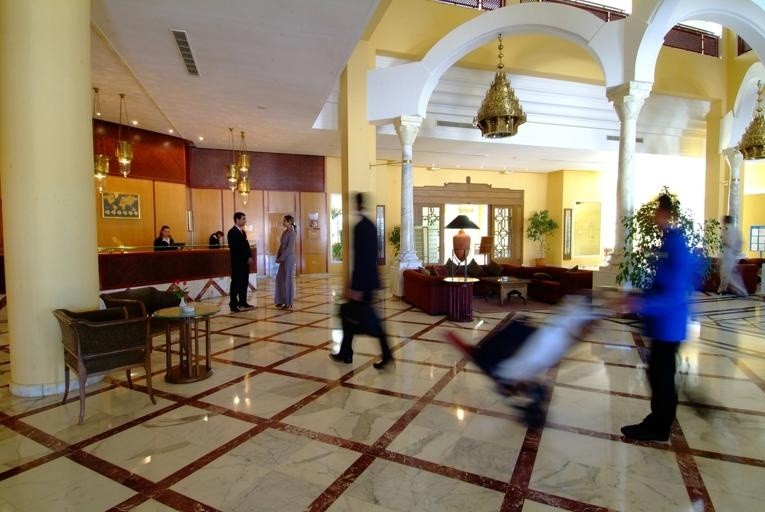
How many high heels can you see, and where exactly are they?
[275,303,286,307]
[279,304,293,310]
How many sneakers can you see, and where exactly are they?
[230,306,241,313]
[620,413,673,442]
[239,303,254,309]
[373,357,396,369]
[329,353,354,365]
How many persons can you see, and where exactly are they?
[154,225,181,251]
[604,195,694,442]
[209,231,223,249]
[716,216,749,298]
[329,192,395,370]
[227,212,254,313]
[275,215,298,309]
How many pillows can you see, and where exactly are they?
[565,264,579,273]
[485,258,503,276]
[444,258,459,275]
[466,258,484,278]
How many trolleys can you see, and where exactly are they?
[440,303,620,429]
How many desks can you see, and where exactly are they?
[442,276,481,321]
[152,302,222,384]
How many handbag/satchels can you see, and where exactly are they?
[338,301,370,330]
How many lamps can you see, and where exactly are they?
[90,88,110,182]
[240,131,249,183]
[471,34,528,140]
[115,92,133,179]
[736,80,765,162]
[443,214,481,261]
[309,212,320,229]
[225,126,239,193]
[237,134,250,204]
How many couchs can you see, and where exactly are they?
[402,263,594,316]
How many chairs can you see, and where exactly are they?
[99,285,192,371]
[695,255,765,296]
[49,306,156,425]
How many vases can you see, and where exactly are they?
[178,295,186,309]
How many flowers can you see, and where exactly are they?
[167,280,189,297]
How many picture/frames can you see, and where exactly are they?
[101,190,141,219]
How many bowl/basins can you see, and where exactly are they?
[183,306,195,313]
[502,277,509,280]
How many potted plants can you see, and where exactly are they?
[526,210,560,267]
